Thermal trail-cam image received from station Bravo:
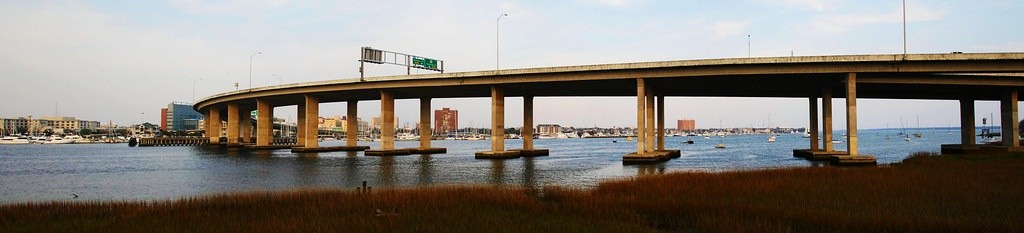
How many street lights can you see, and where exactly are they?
[192,78,202,103]
[495,11,508,69]
[250,50,262,88]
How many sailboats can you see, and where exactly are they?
[360,119,846,152]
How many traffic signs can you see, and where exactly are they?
[423,58,437,70]
[413,58,424,65]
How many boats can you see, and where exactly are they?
[0,132,142,145]
